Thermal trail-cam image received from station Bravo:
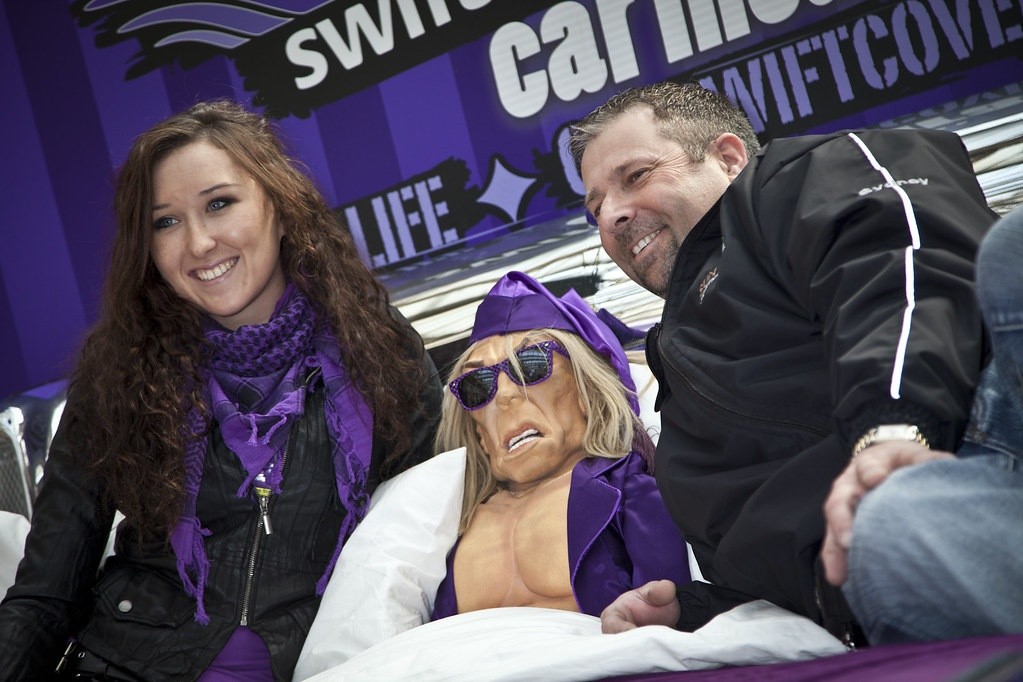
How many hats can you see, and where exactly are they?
[468,272,651,419]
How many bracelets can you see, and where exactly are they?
[854,424,927,460]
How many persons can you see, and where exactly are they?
[566,82,1022,638]
[430,271,689,623]
[0,101,442,682]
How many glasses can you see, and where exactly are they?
[448,339,571,411]
